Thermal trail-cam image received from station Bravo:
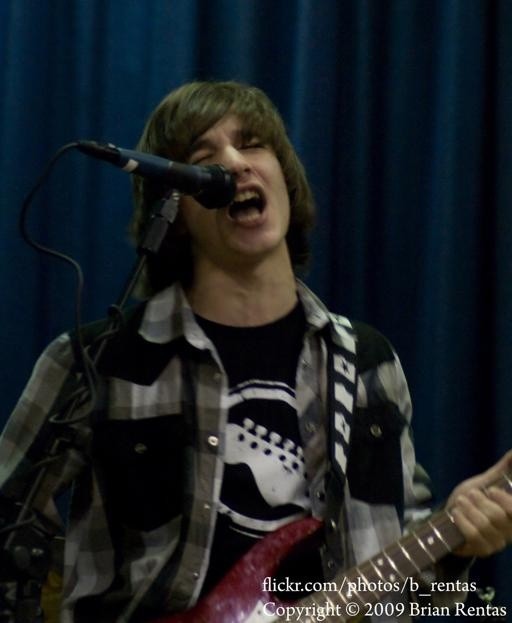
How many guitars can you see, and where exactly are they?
[147,456,511,621]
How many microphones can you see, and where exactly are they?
[76,137,239,209]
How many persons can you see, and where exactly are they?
[1,81,511,623]
